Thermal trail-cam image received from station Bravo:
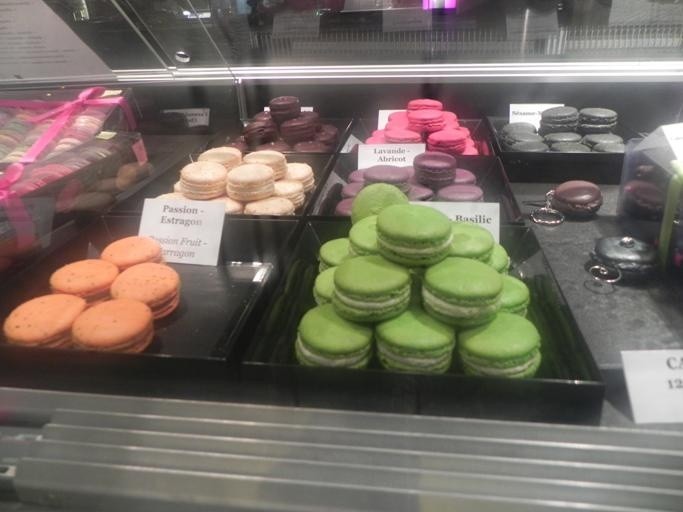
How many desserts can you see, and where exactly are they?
[294,182,540,378]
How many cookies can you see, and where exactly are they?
[117,162,156,184]
[111,263,180,320]
[365,98,481,156]
[156,146,313,215]
[11,141,118,194]
[335,152,483,214]
[49,259,118,305]
[74,190,116,212]
[3,293,84,346]
[72,300,153,353]
[230,95,339,151]
[0,103,109,162]
[502,107,626,154]
[100,236,164,272]
